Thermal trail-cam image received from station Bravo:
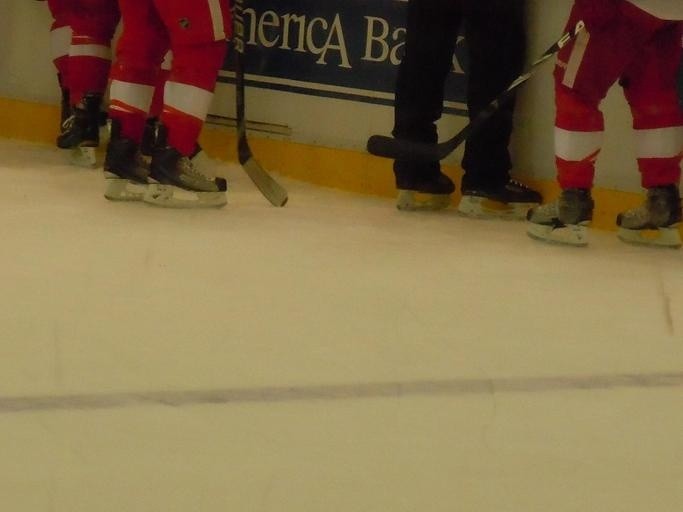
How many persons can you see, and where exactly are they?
[391,0,546,204]
[108,1,233,193]
[47,1,173,152]
[526,1,683,229]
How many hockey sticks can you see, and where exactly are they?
[366,20,584,160]
[235,0,288,207]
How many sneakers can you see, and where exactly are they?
[396,170,593,225]
[617,182,683,230]
[56,92,226,193]
